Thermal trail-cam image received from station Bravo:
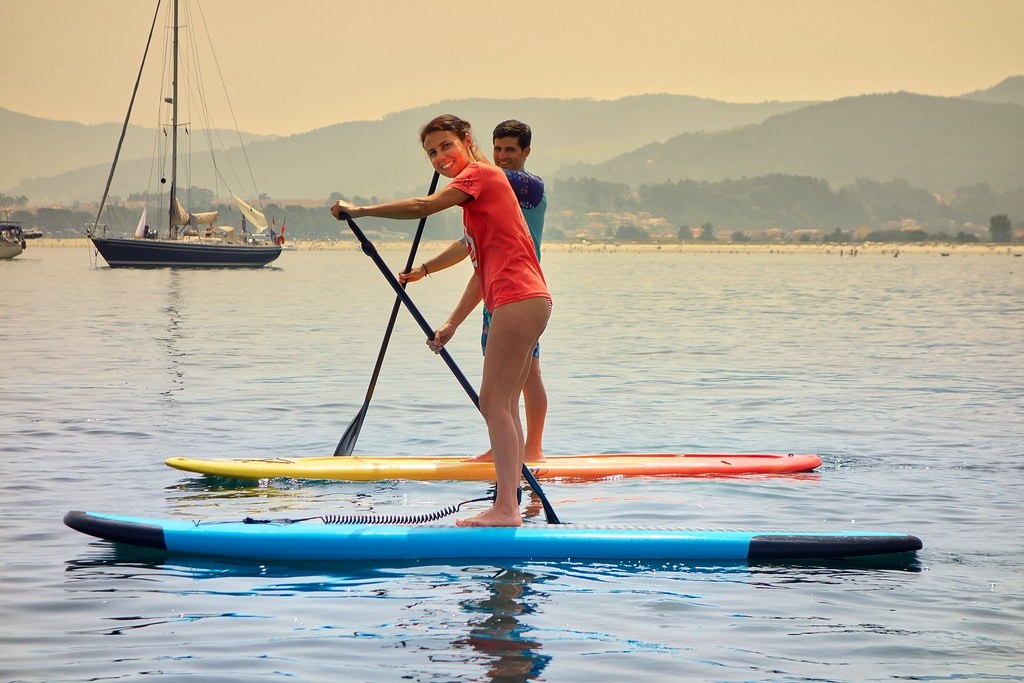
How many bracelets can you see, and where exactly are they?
[422,263,430,279]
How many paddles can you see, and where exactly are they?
[331,167,441,458]
[343,214,562,525]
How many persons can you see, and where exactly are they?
[398,119,548,462]
[329,114,553,527]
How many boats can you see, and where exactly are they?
[0,221,43,258]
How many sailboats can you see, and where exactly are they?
[86,0,283,268]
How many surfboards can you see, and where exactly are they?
[162,451,825,485]
[61,502,926,568]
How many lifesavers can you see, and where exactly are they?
[276,234,285,245]
